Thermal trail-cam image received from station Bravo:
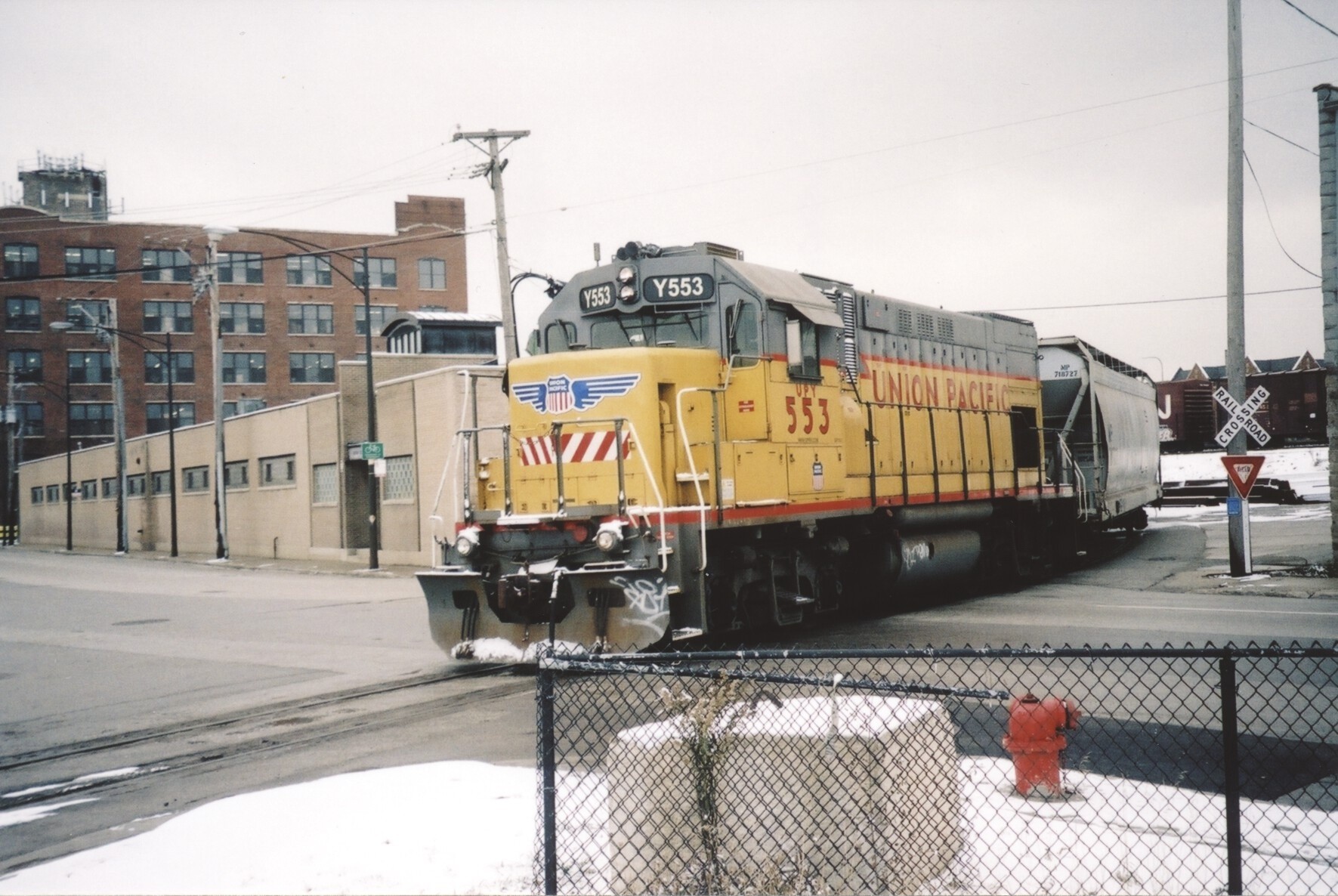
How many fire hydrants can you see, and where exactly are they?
[1002,693,1083,801]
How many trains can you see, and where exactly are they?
[415,238,1164,674]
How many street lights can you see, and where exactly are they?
[201,224,381,569]
[50,321,178,557]
[16,376,73,551]
